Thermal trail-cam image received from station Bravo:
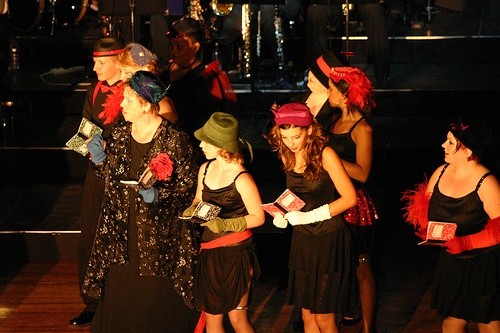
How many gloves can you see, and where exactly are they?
[272,212,288,229]
[284,203,331,225]
[201,216,248,235]
[440,216,500,254]
[183,198,201,217]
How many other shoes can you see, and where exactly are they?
[341,315,361,325]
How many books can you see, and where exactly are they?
[66,118,103,157]
[418,222,458,245]
[119,166,157,191]
[179,201,221,228]
[259,189,306,218]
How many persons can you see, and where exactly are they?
[0,0,500,333]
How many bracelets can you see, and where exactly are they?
[83,4,89,7]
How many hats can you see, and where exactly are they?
[92,38,127,57]
[448,119,491,160]
[329,65,373,110]
[168,17,202,38]
[194,113,240,153]
[129,72,172,104]
[309,50,344,89]
[274,103,313,127]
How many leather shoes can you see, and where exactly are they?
[70,309,95,327]
